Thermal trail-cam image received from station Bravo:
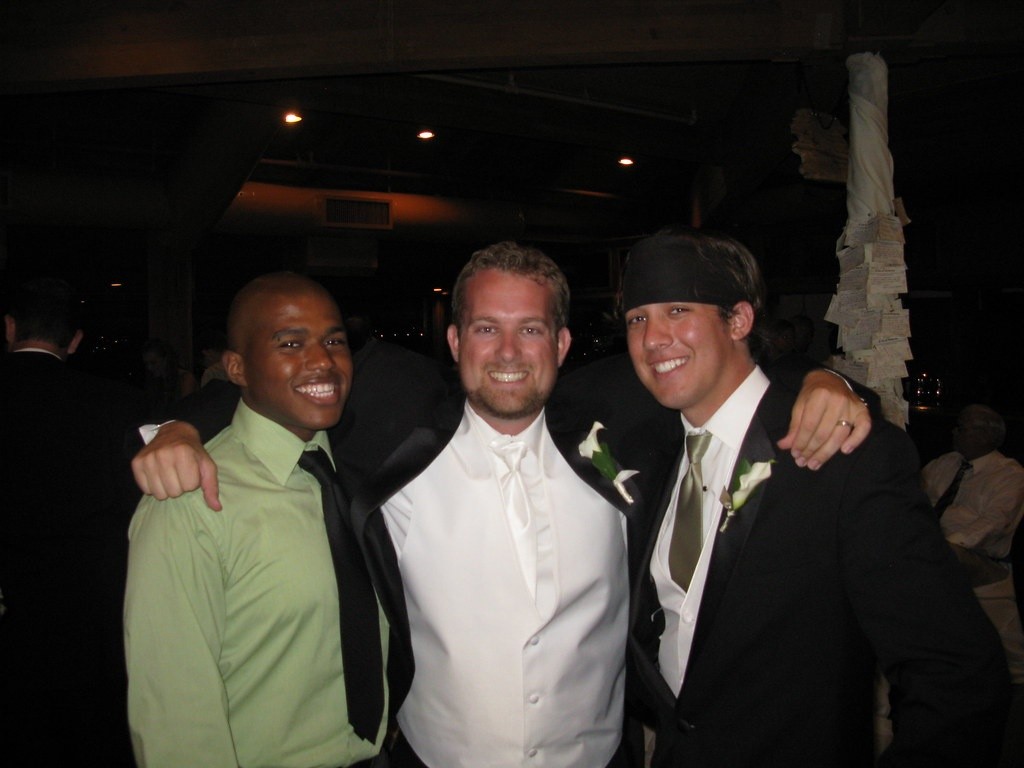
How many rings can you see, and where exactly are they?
[836,420,849,426]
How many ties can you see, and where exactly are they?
[492,442,539,607]
[667,430,713,596]
[299,446,385,747]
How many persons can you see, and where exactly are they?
[919,403,1024,587]
[0,254,137,768]
[122,230,1015,768]
